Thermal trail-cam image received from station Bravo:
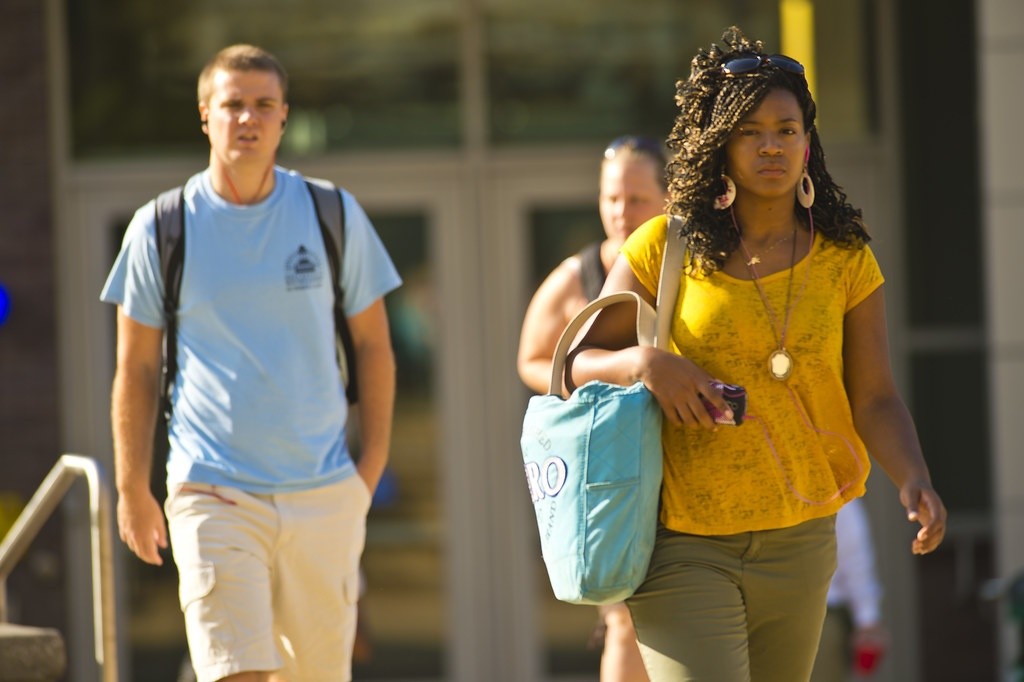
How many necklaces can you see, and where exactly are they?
[735,229,798,266]
[729,225,800,382]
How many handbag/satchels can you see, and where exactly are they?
[516,382,664,609]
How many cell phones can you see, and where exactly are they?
[675,377,746,425]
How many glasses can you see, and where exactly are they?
[716,51,807,82]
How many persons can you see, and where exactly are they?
[560,48,949,682]
[514,125,680,682]
[810,495,895,682]
[91,40,407,682]
